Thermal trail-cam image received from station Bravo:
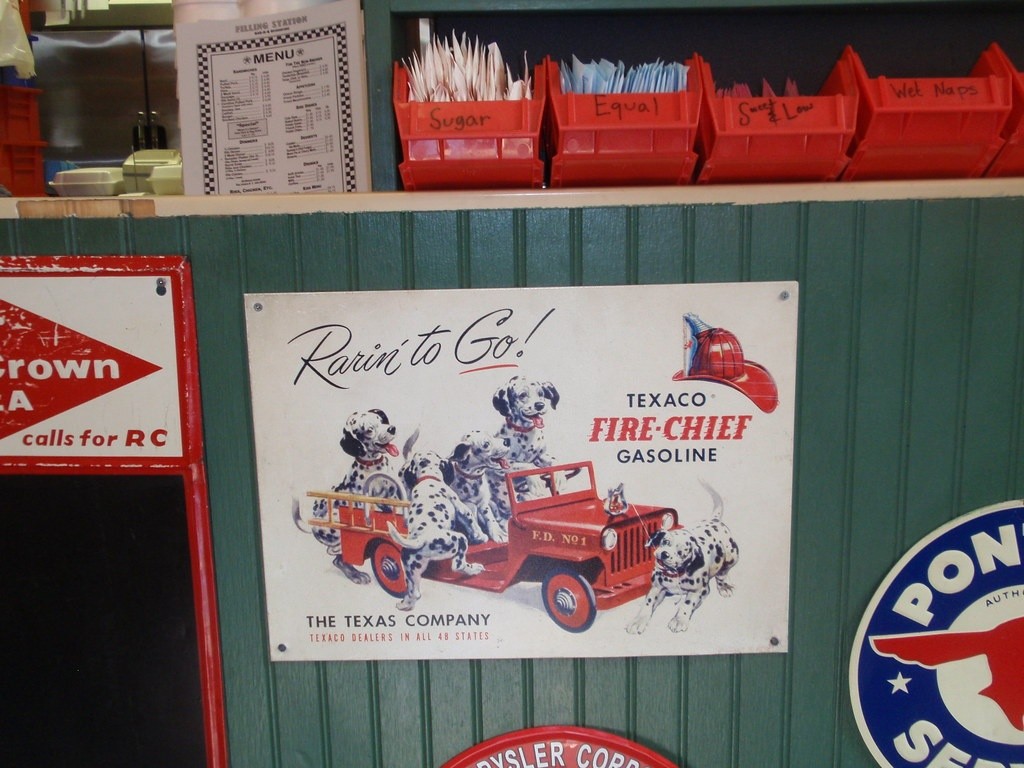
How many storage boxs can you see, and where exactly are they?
[394,39,1024,189]
[0,35,50,197]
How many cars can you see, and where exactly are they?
[345,460,684,631]
